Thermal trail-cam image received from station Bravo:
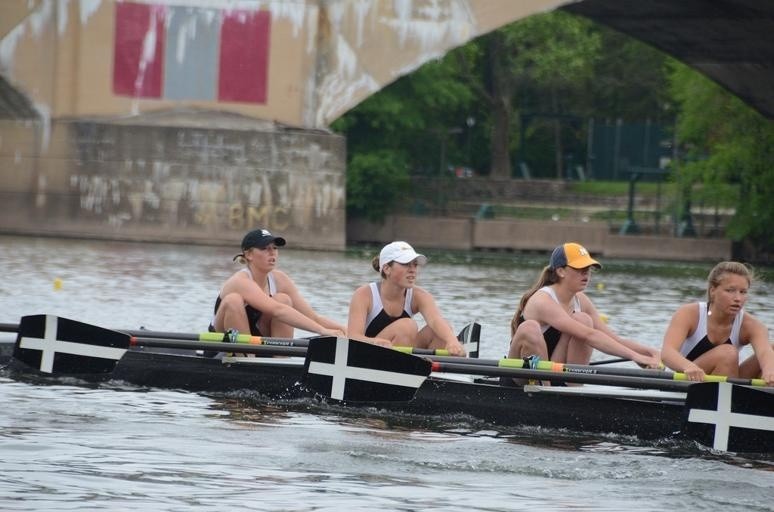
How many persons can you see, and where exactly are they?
[347,241,465,364]
[661,261,774,387]
[499,242,665,389]
[202,228,348,360]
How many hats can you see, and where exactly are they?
[548,240,602,275]
[375,239,428,273]
[240,227,287,251]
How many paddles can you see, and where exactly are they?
[304,339,706,405]
[252,323,480,358]
[14,315,309,374]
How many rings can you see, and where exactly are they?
[691,377,695,379]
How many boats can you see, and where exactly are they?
[0,316,774,457]
